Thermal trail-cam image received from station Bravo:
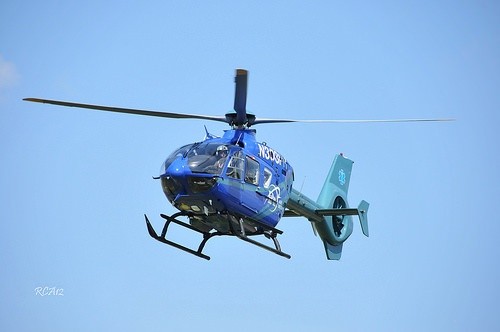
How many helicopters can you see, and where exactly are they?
[22,69,456,260]
